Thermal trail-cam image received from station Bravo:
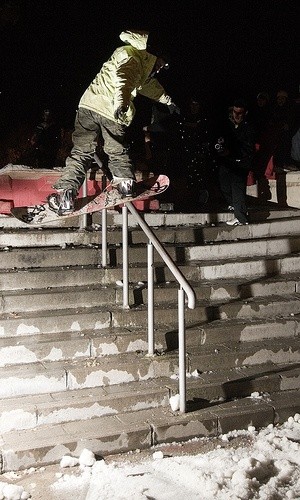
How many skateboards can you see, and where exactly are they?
[10,173,170,225]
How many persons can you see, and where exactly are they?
[254,88,271,110]
[212,99,256,224]
[59,29,177,211]
[257,88,300,208]
[178,91,220,211]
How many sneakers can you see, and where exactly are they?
[103,178,136,199]
[225,216,249,225]
[48,189,77,214]
